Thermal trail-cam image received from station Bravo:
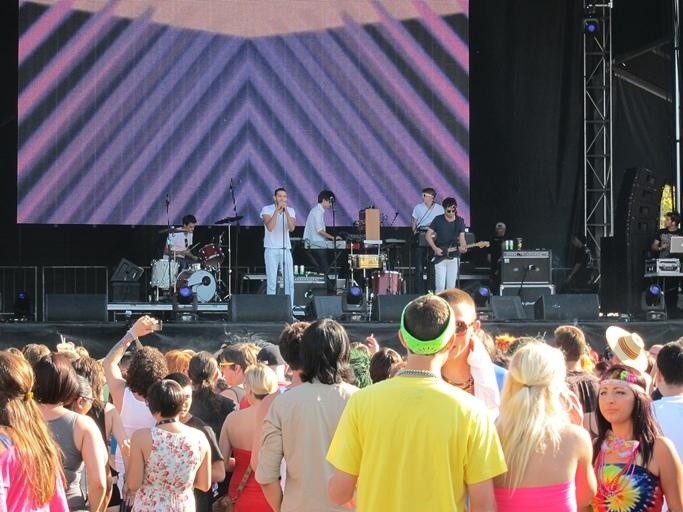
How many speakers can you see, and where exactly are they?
[377,294,423,322]
[44,293,107,323]
[533,294,599,321]
[499,251,555,321]
[303,296,366,323]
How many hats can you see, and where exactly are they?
[258,347,284,365]
[495,222,507,229]
[605,326,649,372]
[647,344,665,360]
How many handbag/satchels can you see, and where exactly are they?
[213,459,252,510]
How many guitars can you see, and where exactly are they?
[431,239,490,265]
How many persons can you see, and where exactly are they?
[557,234,597,290]
[219,362,279,512]
[477,328,508,393]
[188,349,236,445]
[411,188,446,295]
[303,190,336,267]
[651,211,683,320]
[165,349,191,375]
[64,374,92,418]
[553,325,600,447]
[254,316,363,512]
[493,341,598,512]
[325,296,509,512]
[259,188,296,310]
[487,223,514,297]
[604,325,652,371]
[251,321,311,496]
[348,332,380,358]
[30,352,106,512]
[650,343,683,512]
[433,288,509,512]
[426,196,468,294]
[346,348,370,387]
[493,333,516,360]
[164,372,227,512]
[0,351,70,512]
[21,344,50,366]
[119,377,213,511]
[580,364,682,512]
[103,315,170,512]
[258,344,287,393]
[506,337,541,356]
[163,214,201,262]
[368,347,402,386]
[71,355,133,512]
[216,345,256,406]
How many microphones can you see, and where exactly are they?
[229,179,234,193]
[428,198,438,210]
[392,211,400,226]
[329,196,335,209]
[165,191,172,205]
[451,202,459,212]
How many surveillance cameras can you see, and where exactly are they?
[582,17,600,35]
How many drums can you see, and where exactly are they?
[151,259,179,289]
[189,263,201,270]
[355,254,382,268]
[198,244,225,270]
[372,270,402,296]
[174,270,217,302]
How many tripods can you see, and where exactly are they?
[227,294,292,322]
[224,224,234,301]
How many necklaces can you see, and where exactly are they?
[398,369,437,377]
[594,439,641,498]
[441,374,474,392]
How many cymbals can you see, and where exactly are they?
[214,215,244,225]
[158,228,188,234]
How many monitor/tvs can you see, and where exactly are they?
[669,236,682,252]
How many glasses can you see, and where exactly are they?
[422,194,433,199]
[455,320,477,335]
[219,362,235,369]
[79,395,95,404]
[445,210,456,213]
